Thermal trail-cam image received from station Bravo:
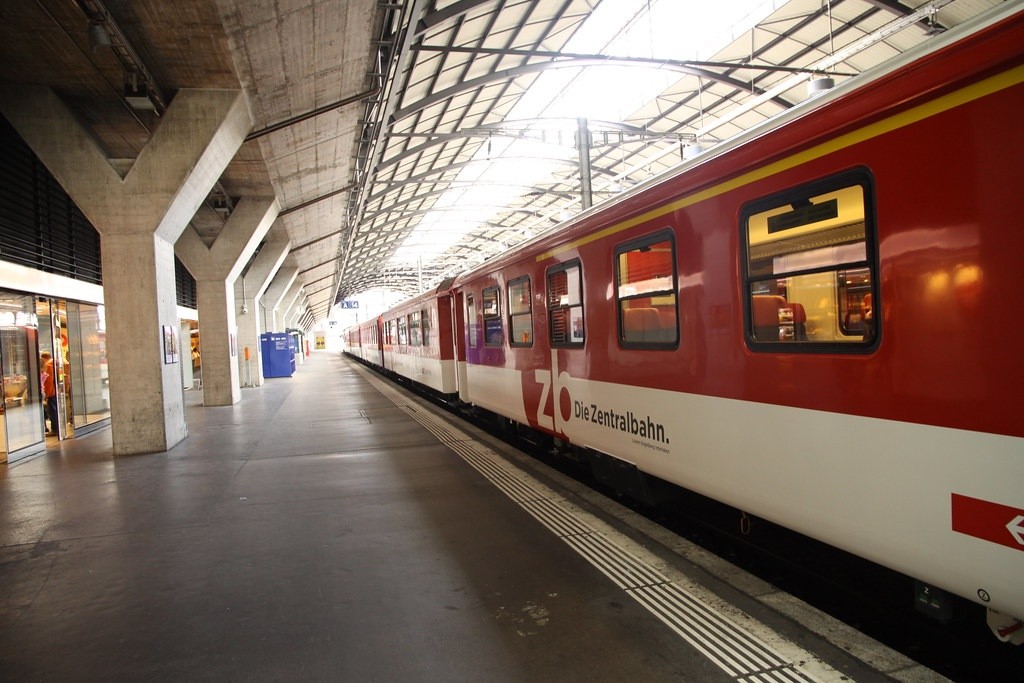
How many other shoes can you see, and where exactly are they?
[46,431,57,437]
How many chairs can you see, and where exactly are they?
[623,307,667,341]
[660,313,677,341]
[788,304,811,341]
[752,294,795,342]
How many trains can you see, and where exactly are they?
[339,0,1024,683]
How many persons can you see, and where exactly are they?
[40,359,50,432]
[192,347,199,360]
[40,351,58,437]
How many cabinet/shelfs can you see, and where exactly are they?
[267,330,297,378]
